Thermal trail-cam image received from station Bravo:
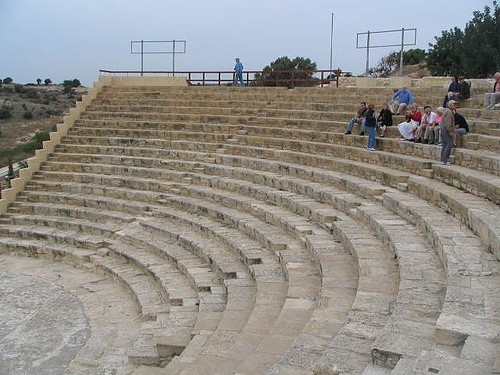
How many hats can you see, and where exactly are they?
[448,100,459,106]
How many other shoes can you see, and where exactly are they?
[391,112,401,115]
[486,105,496,110]
[367,147,375,151]
[345,131,351,134]
[379,135,385,137]
[395,137,443,146]
[359,131,365,136]
[441,161,451,165]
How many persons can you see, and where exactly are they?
[365,105,377,150]
[344,76,471,146]
[440,100,458,164]
[234,58,245,86]
[483,72,500,110]
